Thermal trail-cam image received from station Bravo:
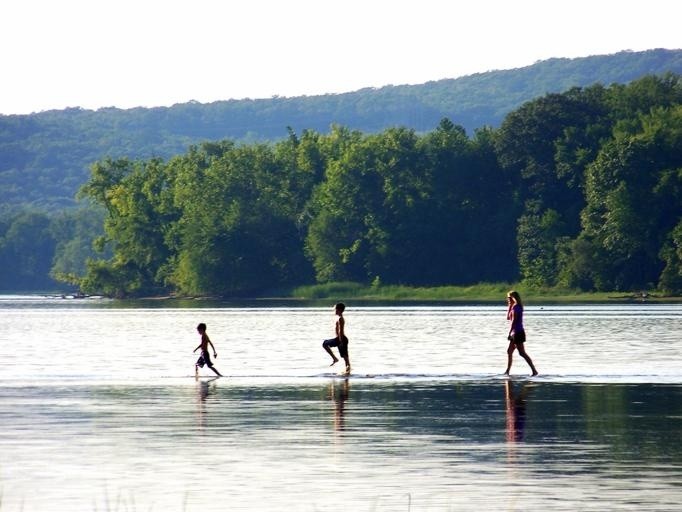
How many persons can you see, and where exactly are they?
[503,291,538,377]
[193,323,222,376]
[322,303,350,374]
